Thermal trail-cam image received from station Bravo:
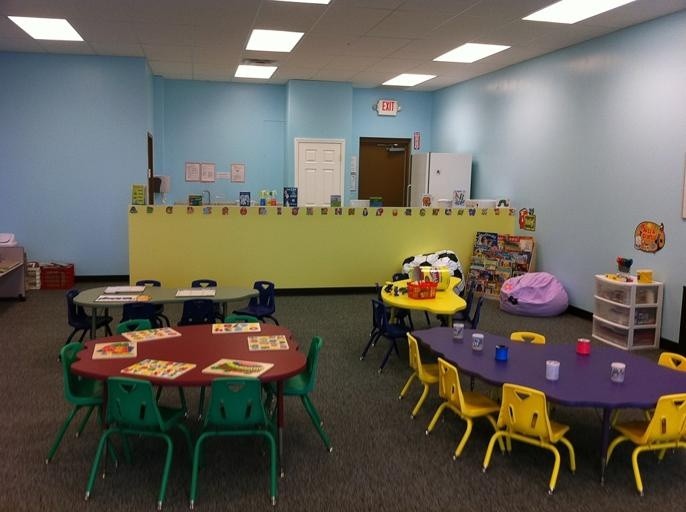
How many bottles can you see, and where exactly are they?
[259,191,277,206]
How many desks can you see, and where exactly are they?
[75,285,259,340]
[69,323,308,480]
[410,326,686,490]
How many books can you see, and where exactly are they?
[95,285,217,302]
[92,320,291,381]
[466,231,533,295]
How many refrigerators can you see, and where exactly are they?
[409,152,474,208]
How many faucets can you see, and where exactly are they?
[202,190,211,204]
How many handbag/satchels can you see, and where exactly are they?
[24,262,41,290]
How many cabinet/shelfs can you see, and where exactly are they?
[0,244,27,302]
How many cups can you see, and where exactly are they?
[545,359,560,382]
[496,344,509,362]
[453,323,465,339]
[577,338,592,354]
[471,333,485,350]
[610,362,626,384]
[637,270,654,283]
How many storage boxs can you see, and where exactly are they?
[27,263,41,292]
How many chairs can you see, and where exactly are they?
[44,341,133,471]
[65,289,113,345]
[136,280,171,328]
[196,314,271,424]
[608,350,686,429]
[120,301,162,333]
[423,357,509,460]
[510,332,552,419]
[480,382,577,496]
[115,319,189,415]
[607,393,686,498]
[189,279,228,314]
[232,280,280,327]
[177,298,217,328]
[358,263,484,375]
[83,377,194,510]
[267,332,335,454]
[187,374,281,511]
[397,331,440,419]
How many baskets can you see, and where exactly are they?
[406,280,439,299]
